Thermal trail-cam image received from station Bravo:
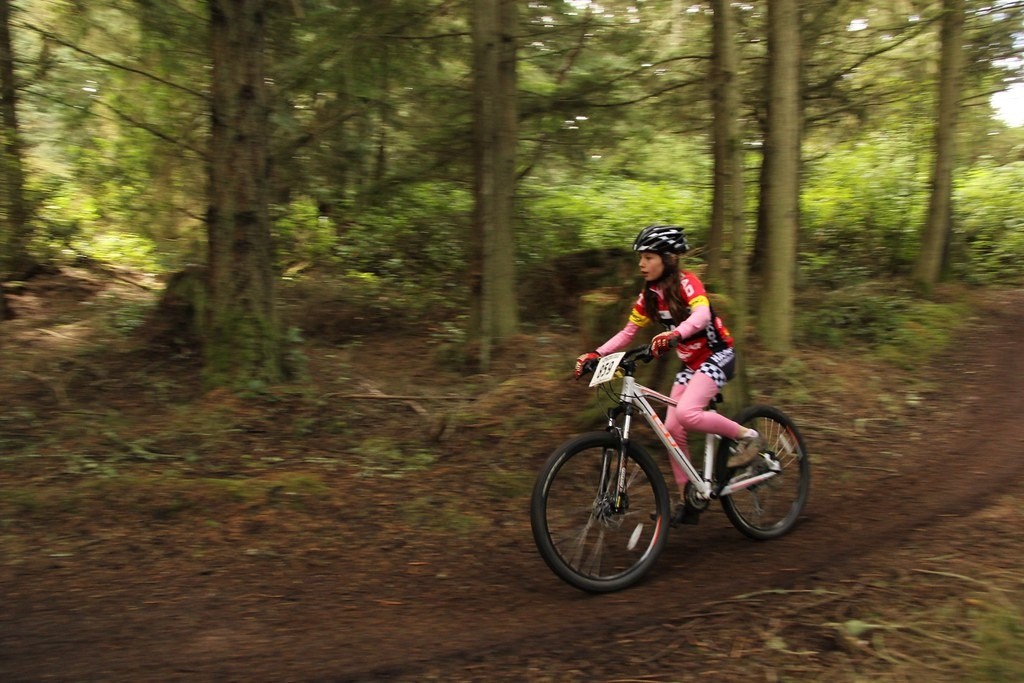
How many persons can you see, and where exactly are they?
[574,224,768,525]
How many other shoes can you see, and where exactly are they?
[725,428,768,469]
[649,500,700,526]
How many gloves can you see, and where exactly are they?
[575,352,599,381]
[651,331,681,361]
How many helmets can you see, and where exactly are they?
[632,225,691,255]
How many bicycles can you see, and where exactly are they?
[530,339,810,595]
